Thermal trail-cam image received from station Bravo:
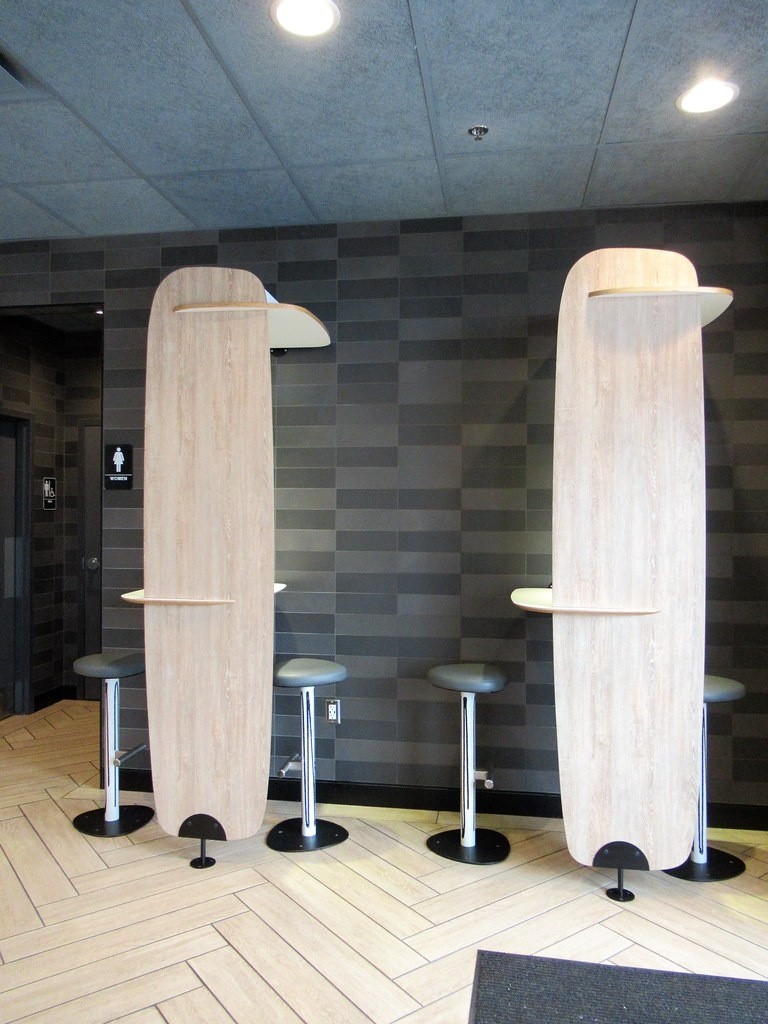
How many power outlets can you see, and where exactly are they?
[476,770,489,780]
[325,699,342,725]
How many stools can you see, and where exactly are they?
[71,651,156,840]
[265,657,350,855]
[425,663,511,866]
[659,674,747,884]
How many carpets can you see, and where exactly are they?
[467,949,768,1024]
